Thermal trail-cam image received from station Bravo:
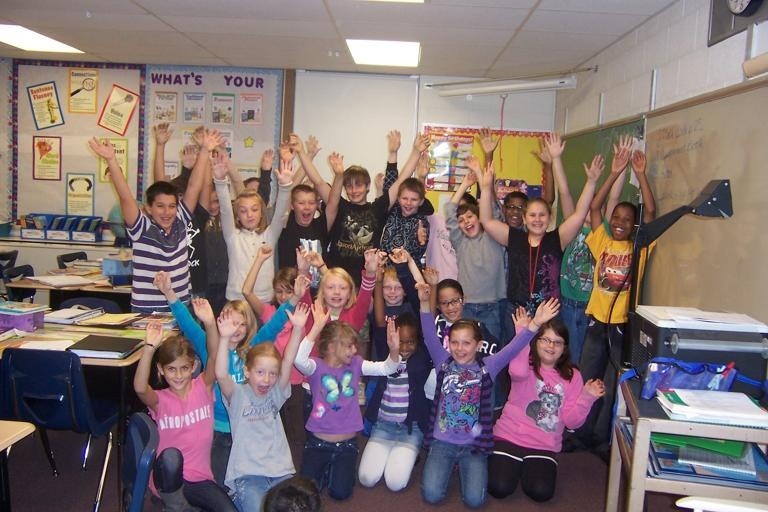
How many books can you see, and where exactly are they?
[0,211,180,361]
[616,305,768,491]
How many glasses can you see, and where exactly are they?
[438,298,463,307]
[535,336,564,347]
[506,204,523,211]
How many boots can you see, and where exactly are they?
[159,484,203,512]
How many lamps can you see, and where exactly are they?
[439,77,577,97]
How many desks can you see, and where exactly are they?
[606,371,768,512]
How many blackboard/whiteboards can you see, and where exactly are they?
[553,117,647,234]
[638,78,768,324]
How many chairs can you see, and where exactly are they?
[0,250,146,512]
[676,496,768,511]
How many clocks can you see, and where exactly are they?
[727,1,761,17]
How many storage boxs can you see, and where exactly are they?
[626,312,768,404]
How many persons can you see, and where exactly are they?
[88,123,657,511]
